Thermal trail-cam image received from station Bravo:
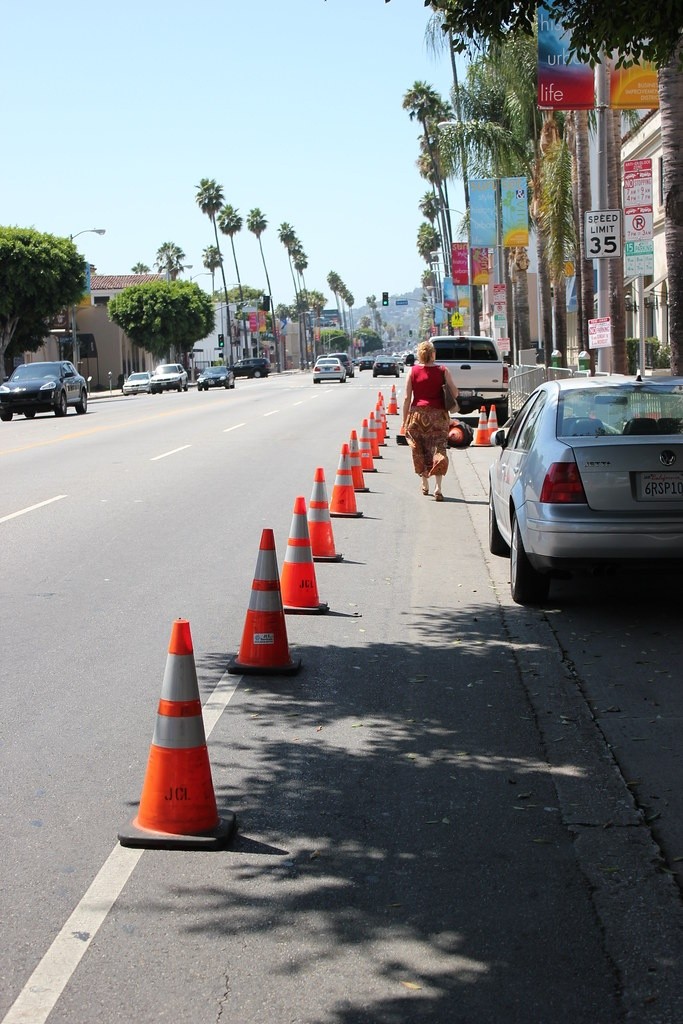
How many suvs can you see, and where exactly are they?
[229,358,271,378]
[149,363,189,394]
[0,361,87,422]
[326,352,356,378]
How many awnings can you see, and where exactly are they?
[594,275,641,311]
[644,274,670,298]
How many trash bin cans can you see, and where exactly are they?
[551,350,562,367]
[578,351,590,371]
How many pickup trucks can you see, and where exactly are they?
[428,335,509,426]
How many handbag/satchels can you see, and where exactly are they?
[440,364,456,411]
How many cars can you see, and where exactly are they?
[489,375,682,605]
[196,366,234,391]
[122,372,153,396]
[372,355,401,378]
[353,353,408,372]
[317,355,327,360]
[312,357,347,384]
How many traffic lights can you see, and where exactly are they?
[218,334,224,347]
[381,291,389,306]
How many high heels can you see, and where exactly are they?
[435,493,443,501]
[421,484,429,494]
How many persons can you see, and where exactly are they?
[400,340,458,501]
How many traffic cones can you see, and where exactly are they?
[279,496,330,615]
[395,424,410,446]
[226,527,302,676]
[306,384,400,563]
[116,617,237,851]
[447,404,498,448]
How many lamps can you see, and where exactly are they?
[624,283,640,300]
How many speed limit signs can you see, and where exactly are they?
[584,209,624,260]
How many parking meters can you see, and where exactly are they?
[108,371,113,394]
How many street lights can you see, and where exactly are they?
[298,311,312,370]
[69,226,108,373]
[166,265,214,382]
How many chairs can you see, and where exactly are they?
[561,417,606,437]
[621,417,658,435]
[656,417,683,434]
